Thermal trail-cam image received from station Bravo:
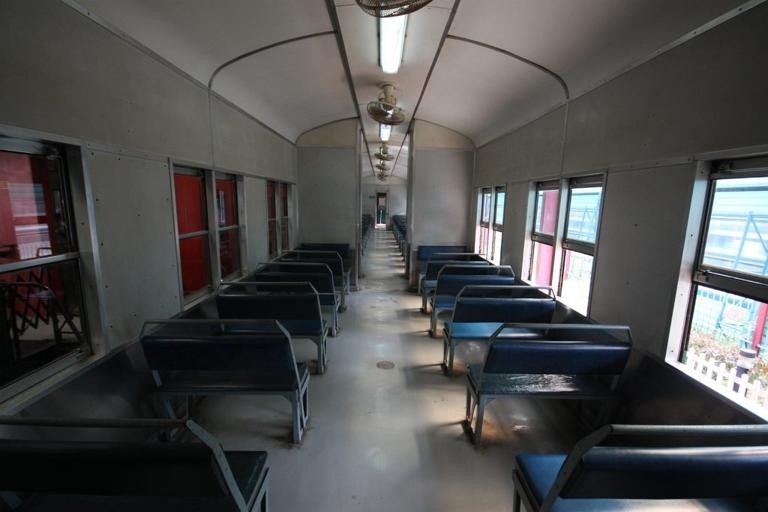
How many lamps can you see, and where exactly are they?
[378,7,412,74]
[379,121,392,141]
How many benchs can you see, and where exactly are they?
[0,415,272,512]
[256,261,343,336]
[460,324,633,445]
[298,241,354,294]
[138,320,313,442]
[414,242,468,295]
[280,249,347,314]
[427,263,515,338]
[439,285,556,376]
[214,281,329,375]
[361,214,374,255]
[392,214,407,261]
[510,425,768,511]
[421,253,497,314]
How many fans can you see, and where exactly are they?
[351,0,435,17]
[376,173,389,182]
[375,161,391,172]
[374,142,394,160]
[368,81,405,125]
